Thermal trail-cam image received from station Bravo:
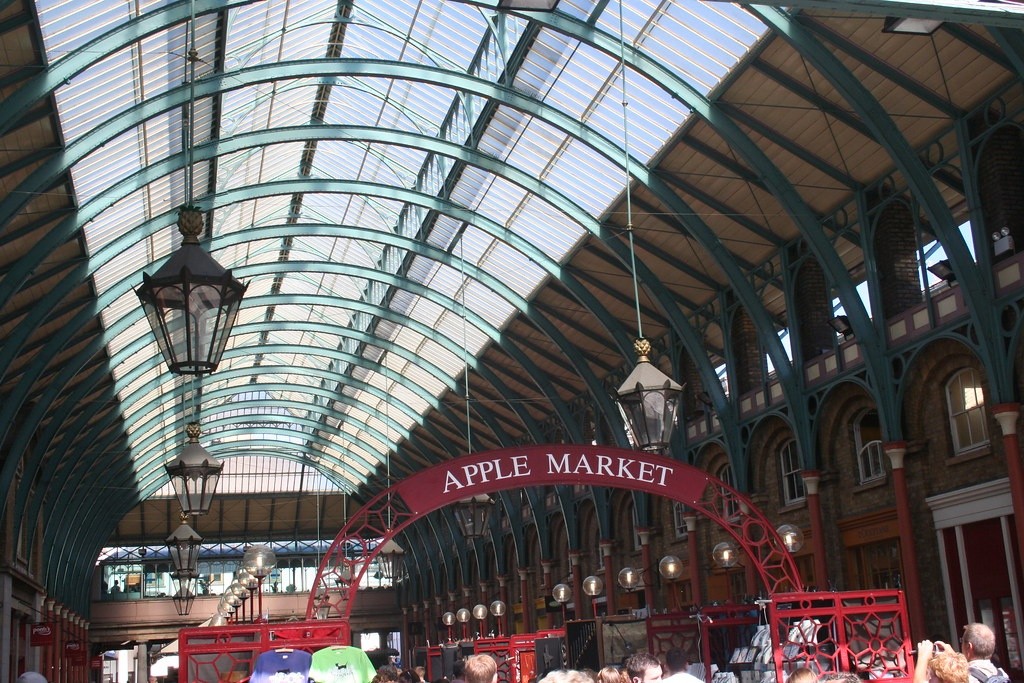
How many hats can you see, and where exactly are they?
[16,671,47,683]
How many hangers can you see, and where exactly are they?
[331,637,346,649]
[274,639,293,653]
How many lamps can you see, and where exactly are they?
[608,622,632,650]
[313,469,331,619]
[138,549,147,555]
[697,392,712,408]
[335,421,356,600]
[132,0,254,373]
[375,571,397,579]
[609,0,689,451]
[618,555,683,615]
[882,16,946,35]
[991,224,1016,261]
[442,601,507,642]
[711,524,804,605]
[449,234,496,539]
[161,374,277,624]
[826,316,855,341]
[496,0,559,12]
[927,258,959,287]
[373,349,408,578]
[553,576,603,623]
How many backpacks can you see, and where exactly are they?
[968,666,1011,683]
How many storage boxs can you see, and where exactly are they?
[728,645,811,662]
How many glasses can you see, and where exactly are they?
[960,637,970,644]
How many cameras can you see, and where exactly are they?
[931,645,939,653]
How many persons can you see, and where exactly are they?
[913,622,1011,683]
[786,668,861,683]
[374,645,705,683]
[102,578,279,595]
